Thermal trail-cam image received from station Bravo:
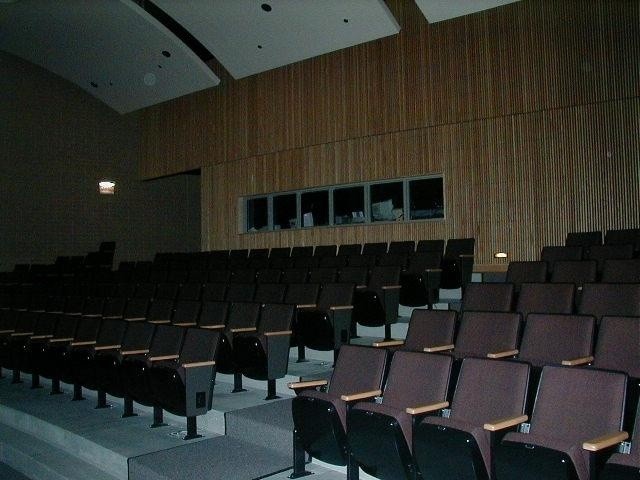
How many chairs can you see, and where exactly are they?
[287,228,640,480]
[2,228,476,441]
